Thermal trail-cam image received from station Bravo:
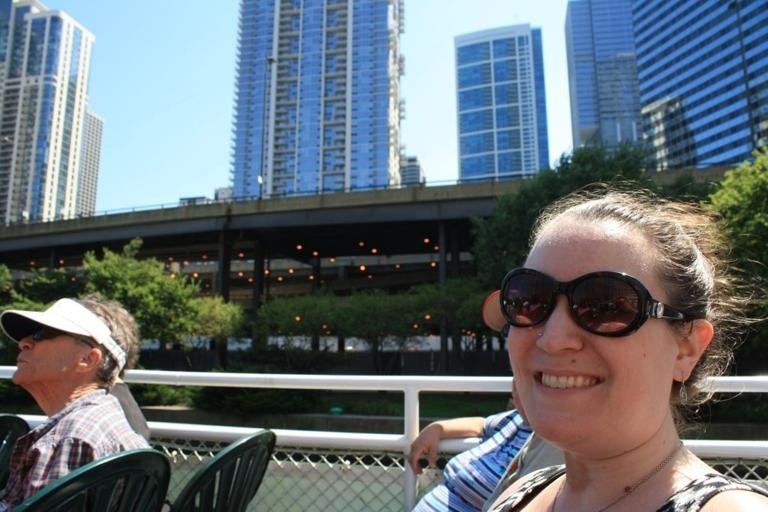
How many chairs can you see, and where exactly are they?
[173,429,277,512]
[11,448,172,512]
[0,412,32,491]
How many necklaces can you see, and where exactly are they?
[551,439,684,512]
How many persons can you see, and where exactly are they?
[403,371,534,511]
[483,183,767,512]
[1,295,160,510]
[107,297,152,444]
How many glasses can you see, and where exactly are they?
[497,265,710,340]
[30,324,95,352]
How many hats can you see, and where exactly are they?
[0,295,128,373]
[481,286,516,337]
